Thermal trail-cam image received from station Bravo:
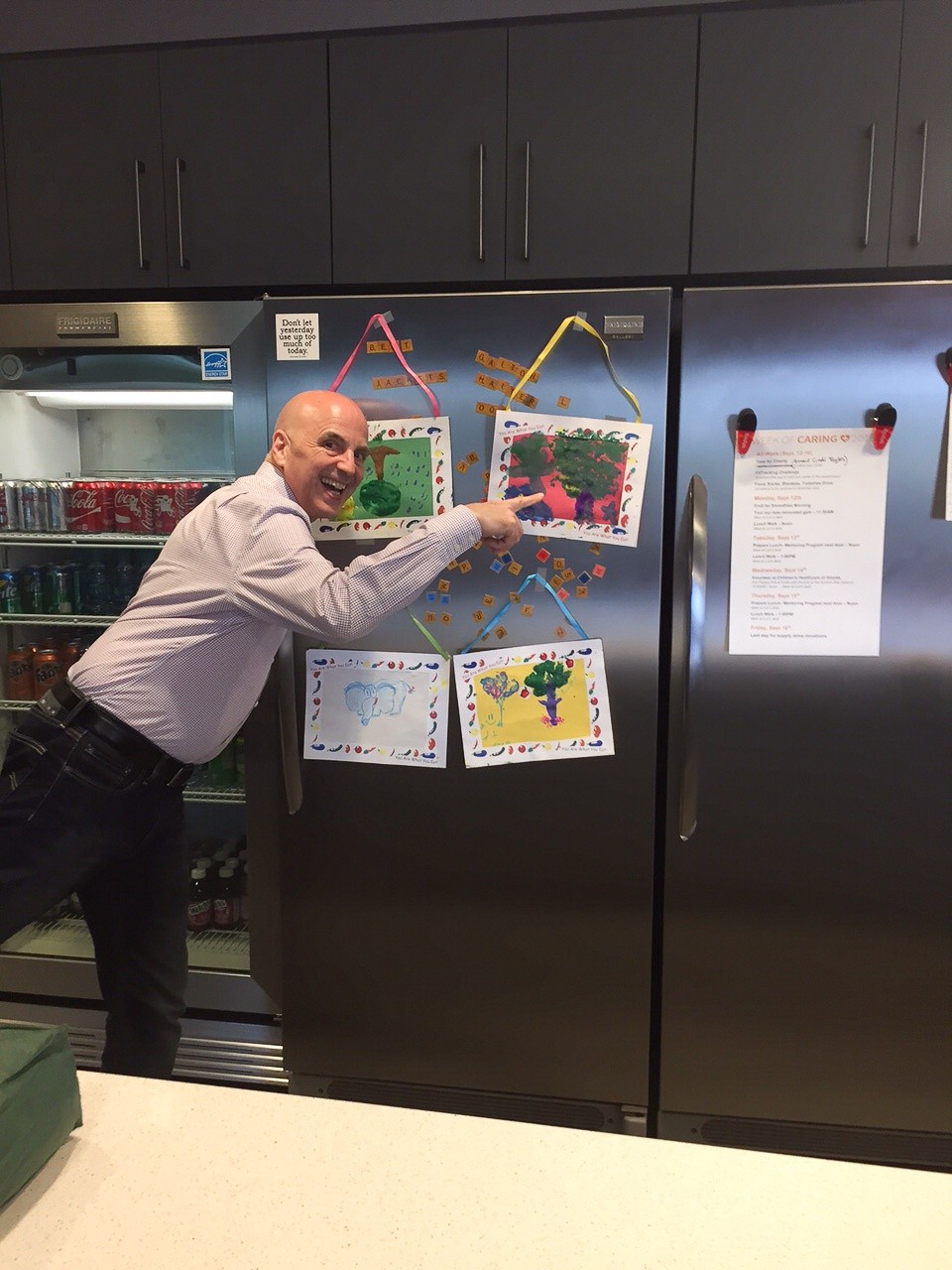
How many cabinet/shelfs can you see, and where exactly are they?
[2,30,332,303]
[331,5,699,289]
[689,0,952,284]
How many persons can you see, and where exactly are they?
[0,391,545,1079]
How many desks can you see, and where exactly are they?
[0,1068,952,1270]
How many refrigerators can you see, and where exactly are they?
[657,280,952,1175]
[0,304,280,1019]
[264,288,670,1138]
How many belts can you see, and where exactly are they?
[51,681,192,790]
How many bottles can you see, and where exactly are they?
[185,833,248,932]
[83,553,157,617]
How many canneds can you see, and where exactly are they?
[0,478,76,532]
[67,478,235,534]
[5,635,93,701]
[0,558,85,613]
